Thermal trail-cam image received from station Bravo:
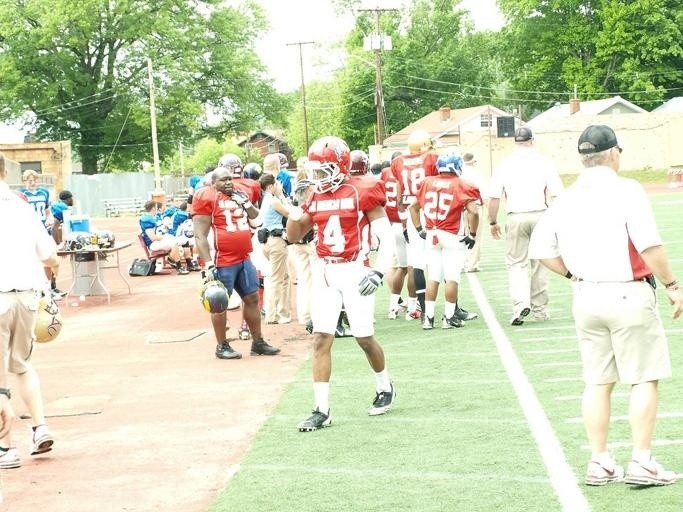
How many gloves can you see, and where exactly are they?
[357,269,385,297]
[291,178,317,208]
[458,231,477,251]
[403,228,411,244]
[415,225,427,240]
[230,189,253,209]
[201,259,219,285]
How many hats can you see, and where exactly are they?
[577,124,623,154]
[514,127,535,143]
[463,152,478,164]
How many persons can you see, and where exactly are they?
[0,157,60,469]
[139,130,485,359]
[18,170,67,296]
[0,345,16,441]
[49,190,75,245]
[527,125,681,491]
[281,135,398,433]
[485,129,565,328]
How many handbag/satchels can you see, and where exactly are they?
[271,228,283,238]
[128,257,157,277]
[257,227,270,244]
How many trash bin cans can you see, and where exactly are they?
[69,219,90,232]
[669,165,683,186]
[152,194,167,213]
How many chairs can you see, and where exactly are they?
[137,232,175,277]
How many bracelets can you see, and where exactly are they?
[489,221,498,228]
[563,270,574,281]
[0,387,13,399]
[661,279,680,288]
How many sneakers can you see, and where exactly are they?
[0,446,25,470]
[47,286,69,302]
[249,337,282,357]
[298,406,333,434]
[166,255,203,277]
[225,300,294,341]
[215,340,243,360]
[624,452,678,488]
[305,310,355,339]
[583,452,628,488]
[28,422,55,458]
[367,379,397,417]
[460,265,481,274]
[385,298,551,332]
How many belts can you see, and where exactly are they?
[0,288,36,294]
[572,275,650,284]
[318,255,354,265]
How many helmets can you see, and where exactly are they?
[306,135,352,179]
[407,128,434,156]
[199,279,231,316]
[274,152,289,168]
[349,149,370,176]
[33,293,64,343]
[217,152,244,180]
[242,162,263,180]
[434,148,465,178]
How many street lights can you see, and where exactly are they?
[148,87,166,188]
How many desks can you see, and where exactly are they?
[53,239,133,305]
